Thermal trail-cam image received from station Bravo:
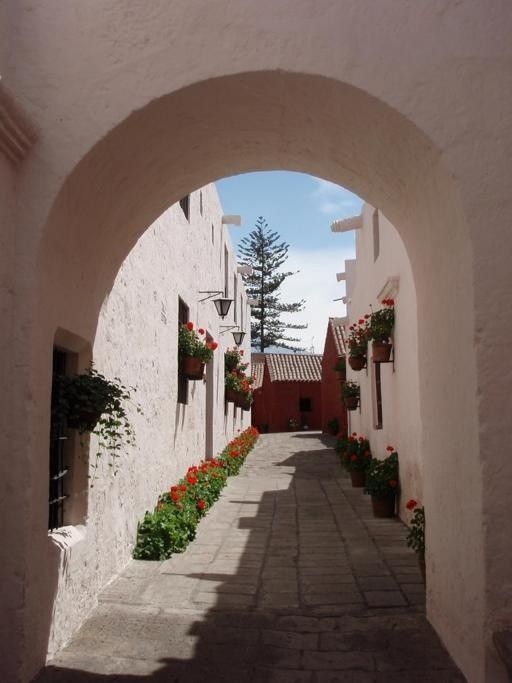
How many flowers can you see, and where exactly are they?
[333,428,426,584]
[224,345,257,411]
[177,320,220,363]
[363,297,395,341]
[342,315,367,356]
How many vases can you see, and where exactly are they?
[373,345,392,361]
[181,354,205,381]
[349,357,365,371]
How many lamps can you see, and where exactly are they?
[218,324,246,347]
[198,288,235,319]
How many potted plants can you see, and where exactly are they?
[338,381,361,411]
[56,359,144,487]
